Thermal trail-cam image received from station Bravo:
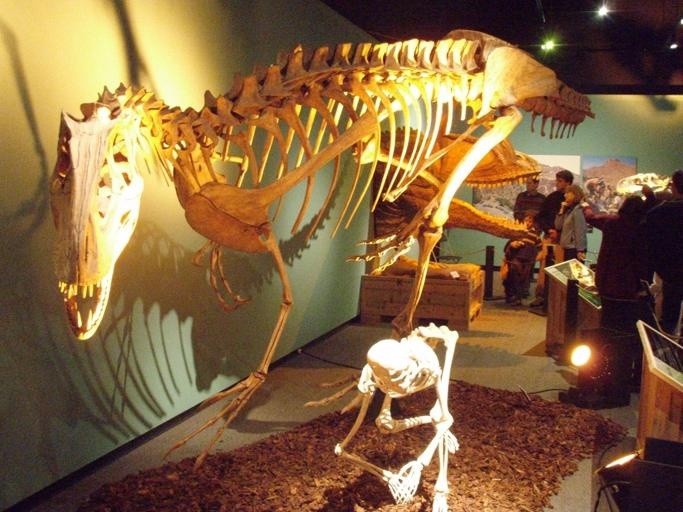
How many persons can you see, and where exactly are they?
[504,170,683,393]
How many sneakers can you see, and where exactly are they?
[503,296,521,310]
[528,297,546,317]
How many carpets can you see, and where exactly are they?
[77,380,628,512]
